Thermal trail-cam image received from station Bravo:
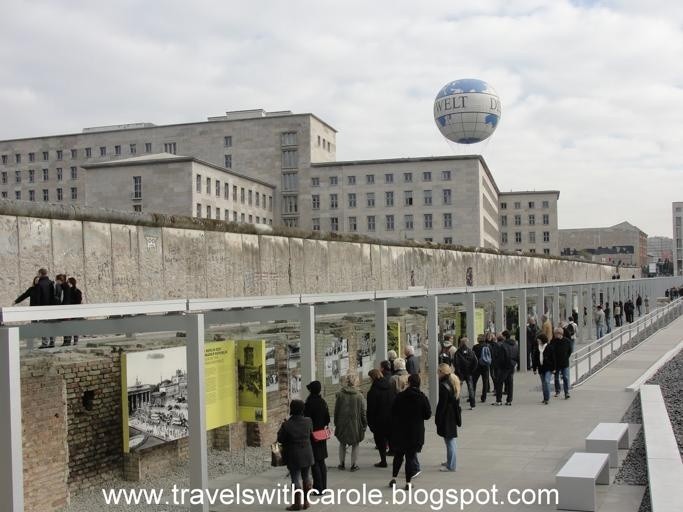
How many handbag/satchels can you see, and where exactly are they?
[457,406,461,427]
[271,442,286,466]
[313,429,331,442]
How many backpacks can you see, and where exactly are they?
[478,344,491,366]
[566,322,574,336]
[439,343,452,364]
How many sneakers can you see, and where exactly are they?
[337,447,456,490]
[542,393,570,404]
[466,389,513,411]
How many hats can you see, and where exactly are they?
[306,381,321,393]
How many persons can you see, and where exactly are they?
[332,373,368,472]
[12,268,83,349]
[664,285,683,301]
[300,380,330,495]
[367,293,651,492]
[277,399,316,511]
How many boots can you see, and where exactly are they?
[287,488,302,510]
[303,484,312,510]
